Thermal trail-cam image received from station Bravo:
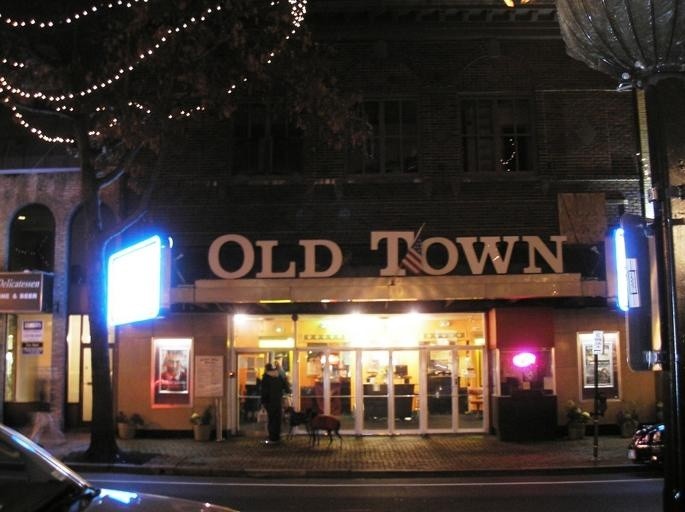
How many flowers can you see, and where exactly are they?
[564,398,593,423]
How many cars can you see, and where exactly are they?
[627,423,667,469]
[0,422,241,511]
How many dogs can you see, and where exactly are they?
[284,406,343,450]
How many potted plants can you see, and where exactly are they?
[189,404,215,442]
[617,397,645,438]
[114,411,146,440]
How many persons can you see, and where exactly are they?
[261,364,291,441]
[162,351,186,391]
[29,378,65,447]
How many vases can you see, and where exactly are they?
[568,422,586,440]
